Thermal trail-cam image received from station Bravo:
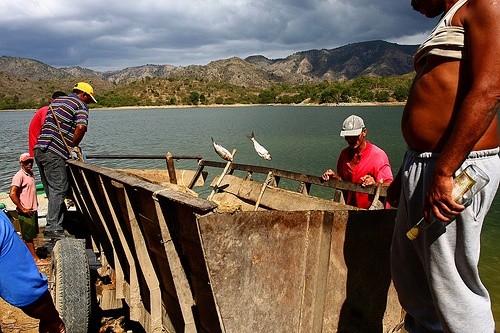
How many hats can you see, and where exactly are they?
[340,115,365,136]
[73,82,97,103]
[20,153,34,162]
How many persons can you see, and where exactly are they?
[386,0,500,332]
[322,115,394,210]
[0,209,66,333]
[28,91,68,163]
[9,152,51,265]
[32,82,97,242]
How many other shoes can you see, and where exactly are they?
[36,259,51,266]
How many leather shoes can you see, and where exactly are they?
[44,230,75,238]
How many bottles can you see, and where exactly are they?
[406,164,490,240]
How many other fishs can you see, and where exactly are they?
[211,136,233,162]
[246,130,272,161]
